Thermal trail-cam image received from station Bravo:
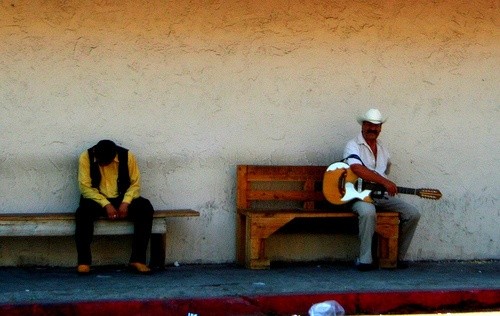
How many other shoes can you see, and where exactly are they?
[129,262,151,273]
[398,260,414,268]
[77,265,89,273]
[358,263,371,271]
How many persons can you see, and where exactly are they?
[342,108,421,269]
[75,139,154,274]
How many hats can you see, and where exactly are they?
[356,108,388,125]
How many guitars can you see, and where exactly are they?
[322,161,442,206]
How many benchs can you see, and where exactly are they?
[0,208,201,270]
[235,165,398,268]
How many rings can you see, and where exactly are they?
[113,214,116,218]
